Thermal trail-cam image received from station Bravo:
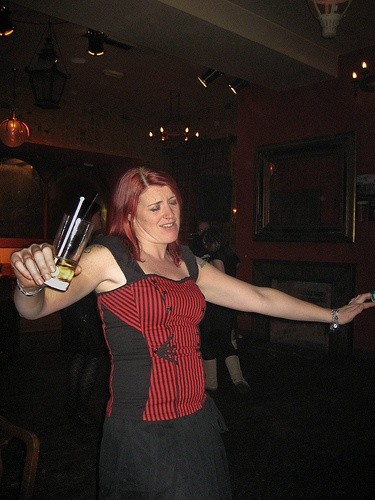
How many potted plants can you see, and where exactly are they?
[0,0,14,36]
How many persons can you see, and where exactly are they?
[11,168,375,499]
[187,217,212,258]
[195,230,253,396]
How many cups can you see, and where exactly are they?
[42,214,94,291]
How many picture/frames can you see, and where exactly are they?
[253,131,356,242]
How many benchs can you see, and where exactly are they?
[0,351,100,500]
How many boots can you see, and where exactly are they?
[202,359,218,390]
[225,355,251,394]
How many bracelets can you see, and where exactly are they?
[15,278,45,297]
[329,308,340,331]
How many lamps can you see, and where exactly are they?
[353,61,375,92]
[198,67,222,88]
[0,68,29,148]
[25,15,69,110]
[149,89,200,153]
[87,28,106,56]
[229,79,247,94]
